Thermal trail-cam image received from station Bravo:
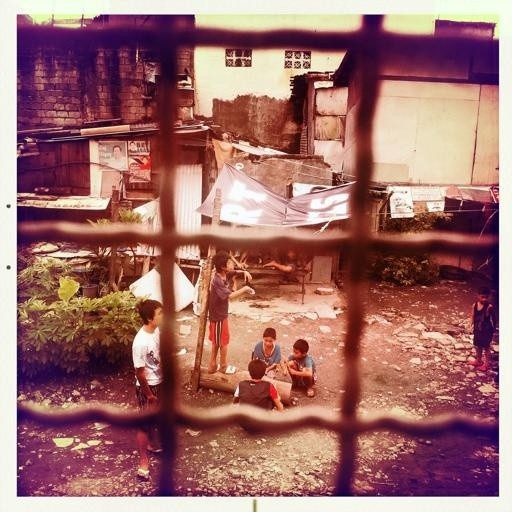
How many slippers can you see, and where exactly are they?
[208,363,240,375]
[307,388,315,398]
[137,444,163,480]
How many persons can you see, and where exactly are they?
[128,142,137,153]
[232,360,284,432]
[207,250,255,374]
[131,299,163,481]
[466,286,496,372]
[284,339,317,397]
[255,327,282,376]
[110,144,127,169]
